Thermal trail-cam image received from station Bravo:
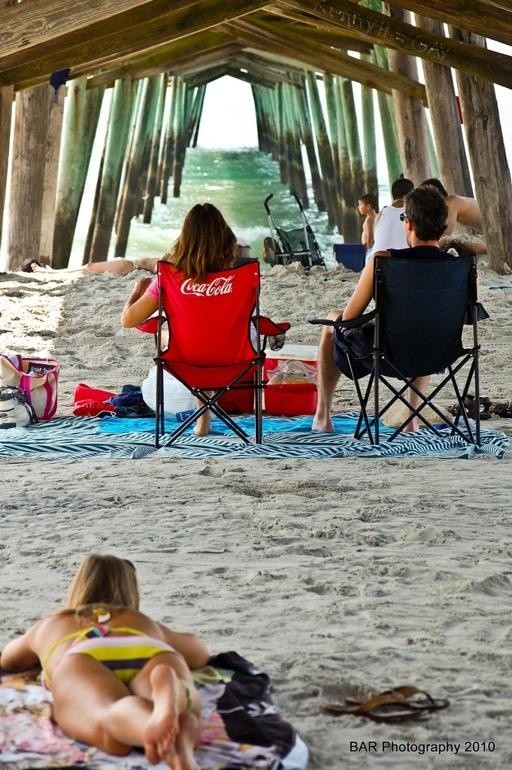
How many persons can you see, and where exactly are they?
[120,204,262,436]
[311,186,457,433]
[356,192,377,250]
[31,257,158,277]
[420,177,486,258]
[0,554,207,770]
[373,178,413,250]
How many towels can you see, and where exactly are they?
[92,410,396,436]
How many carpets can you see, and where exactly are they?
[1,639,316,770]
[2,410,504,463]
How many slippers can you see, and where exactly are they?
[325,686,449,721]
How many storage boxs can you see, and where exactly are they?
[261,343,321,415]
[211,361,258,416]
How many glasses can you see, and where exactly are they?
[401,213,410,221]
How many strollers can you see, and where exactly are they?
[263,190,329,273]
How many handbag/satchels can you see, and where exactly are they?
[0,354,58,429]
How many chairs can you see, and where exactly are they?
[309,248,486,448]
[138,256,292,451]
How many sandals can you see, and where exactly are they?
[447,395,492,420]
